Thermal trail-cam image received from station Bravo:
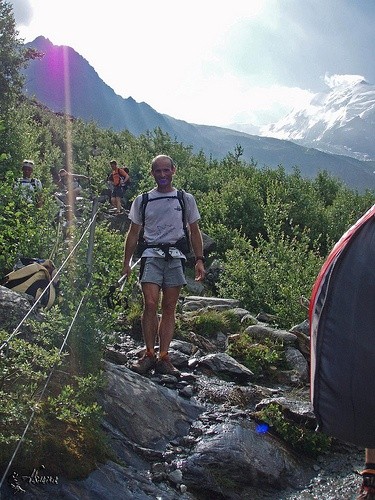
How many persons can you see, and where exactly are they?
[107,160,128,212]
[13,159,43,207]
[119,155,206,374]
[58,170,89,190]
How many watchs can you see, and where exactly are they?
[195,256,205,263]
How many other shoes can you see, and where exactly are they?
[131,353,157,373]
[155,356,181,377]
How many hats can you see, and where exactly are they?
[110,160,116,165]
[22,160,35,168]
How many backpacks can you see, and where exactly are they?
[117,167,131,190]
[308,206,375,446]
[3,257,60,310]
